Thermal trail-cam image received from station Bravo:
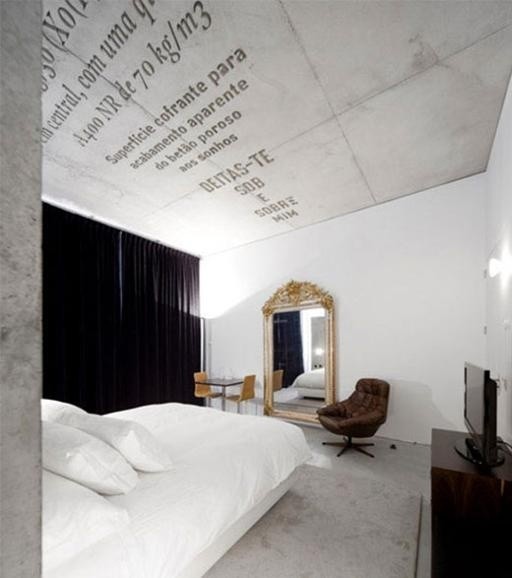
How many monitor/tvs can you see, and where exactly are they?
[455,362,505,469]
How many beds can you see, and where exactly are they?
[293,365,326,400]
[39,399,305,578]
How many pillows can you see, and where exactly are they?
[40,395,170,565]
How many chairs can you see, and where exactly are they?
[227,374,256,412]
[192,372,222,405]
[272,369,284,392]
[317,378,390,457]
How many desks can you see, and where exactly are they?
[430,423,512,575]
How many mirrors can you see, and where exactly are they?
[262,279,336,427]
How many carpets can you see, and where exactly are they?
[203,455,425,578]
